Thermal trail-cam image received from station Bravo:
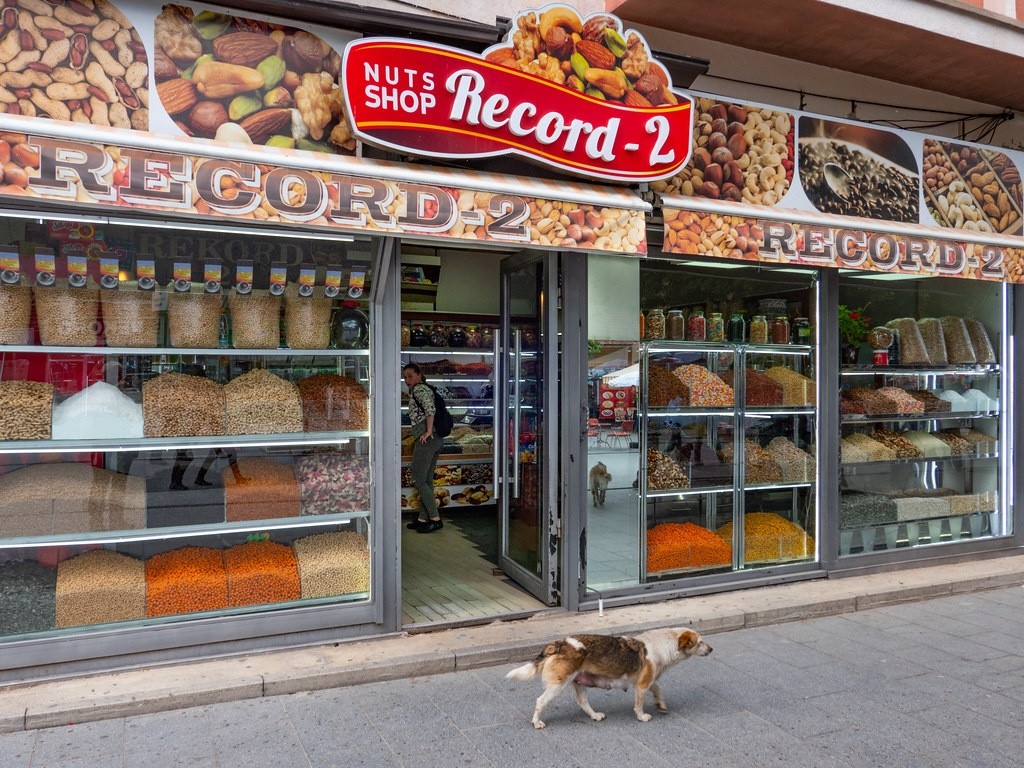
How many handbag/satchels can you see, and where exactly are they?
[412,383,453,438]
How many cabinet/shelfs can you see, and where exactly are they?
[0,343,369,642]
[639,339,815,580]
[401,346,563,476]
[837,364,1002,530]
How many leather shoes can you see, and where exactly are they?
[417,519,443,533]
[407,519,422,529]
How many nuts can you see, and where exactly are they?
[0,0,150,133]
[648,94,797,205]
[0,132,648,257]
[921,138,1023,235]
[152,2,360,156]
[660,209,1024,284]
[400,485,495,510]
[486,6,680,110]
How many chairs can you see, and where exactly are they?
[587,418,636,450]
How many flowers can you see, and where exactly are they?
[838,299,874,342]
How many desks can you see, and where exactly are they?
[590,423,612,450]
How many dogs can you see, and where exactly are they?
[589,460,612,508]
[504,625,713,730]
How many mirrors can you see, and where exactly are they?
[587,340,640,589]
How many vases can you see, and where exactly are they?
[843,344,862,365]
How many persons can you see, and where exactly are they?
[194,448,252,486]
[403,363,444,532]
[168,449,194,491]
[663,396,683,455]
[694,423,730,465]
[88,361,139,531]
